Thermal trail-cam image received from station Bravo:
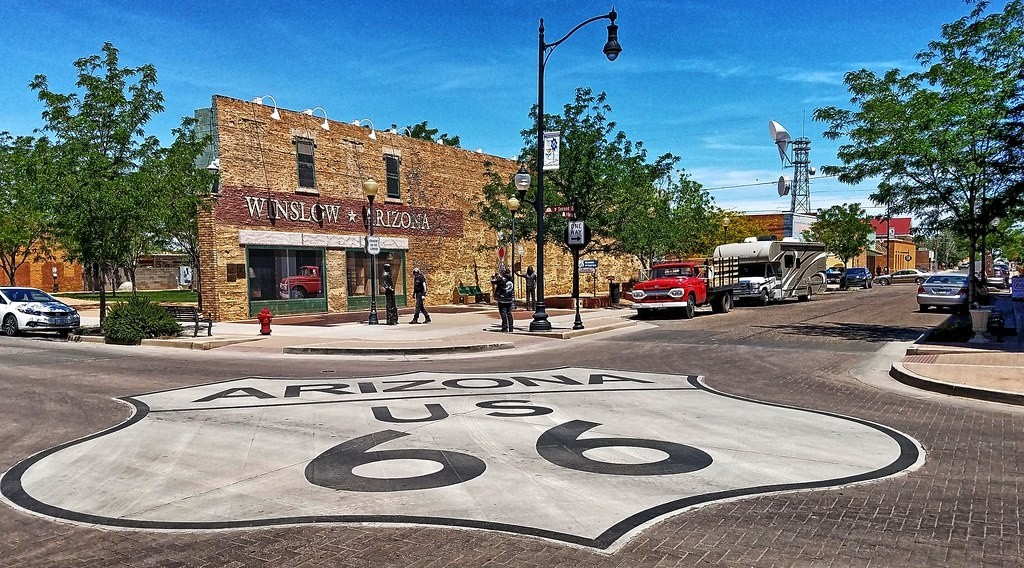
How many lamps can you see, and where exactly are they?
[305,107,330,130]
[352,117,376,140]
[206,159,219,169]
[253,95,280,120]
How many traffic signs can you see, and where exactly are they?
[567,220,585,245]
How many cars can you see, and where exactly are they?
[872,268,934,286]
[916,273,984,313]
[0,286,81,337]
[958,257,1013,289]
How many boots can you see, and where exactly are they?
[423,315,431,323]
[409,316,418,323]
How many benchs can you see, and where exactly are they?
[162,306,213,336]
[972,301,1004,343]
[451,285,490,304]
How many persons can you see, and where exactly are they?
[409,267,431,324]
[491,269,513,333]
[382,264,394,291]
[514,266,536,311]
[1005,262,1024,332]
[876,265,882,276]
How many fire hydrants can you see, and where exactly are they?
[257,307,273,335]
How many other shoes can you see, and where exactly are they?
[527,308,533,311]
[532,308,536,311]
[500,329,508,333]
[509,327,513,332]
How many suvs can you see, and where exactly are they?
[839,267,873,290]
[826,266,845,282]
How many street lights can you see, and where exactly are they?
[363,175,379,325]
[506,193,521,309]
[513,3,622,332]
[721,215,731,245]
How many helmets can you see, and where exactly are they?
[413,268,419,275]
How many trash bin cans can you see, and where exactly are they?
[609,283,619,303]
[178,266,193,286]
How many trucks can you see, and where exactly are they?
[279,265,322,299]
[630,255,740,320]
[712,235,827,306]
